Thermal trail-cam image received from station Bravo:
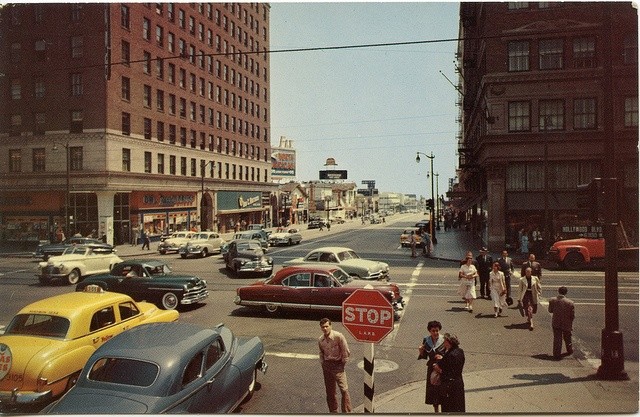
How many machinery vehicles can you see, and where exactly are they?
[548,219,638,269]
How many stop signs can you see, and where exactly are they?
[342,288,395,344]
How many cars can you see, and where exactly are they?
[282,247,391,281]
[268,227,303,246]
[179,231,227,258]
[400,227,425,247]
[36,236,114,260]
[34,243,125,285]
[225,238,275,277]
[0,284,180,412]
[332,216,346,221]
[234,264,406,320]
[75,258,210,310]
[308,217,323,228]
[320,217,331,226]
[36,320,268,414]
[412,219,430,233]
[233,223,271,239]
[157,231,195,254]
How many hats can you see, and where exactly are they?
[479,246,489,253]
[443,333,460,347]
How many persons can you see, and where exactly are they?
[132,226,150,251]
[443,209,463,232]
[319,221,330,232]
[548,286,575,360]
[126,266,146,278]
[315,276,323,287]
[420,229,429,256]
[459,257,478,312]
[518,226,563,254]
[417,320,448,413]
[460,252,477,286]
[489,262,507,318]
[521,254,541,281]
[317,318,351,413]
[432,333,465,412]
[518,267,542,331]
[410,231,416,258]
[55,226,107,244]
[495,250,514,297]
[476,247,493,301]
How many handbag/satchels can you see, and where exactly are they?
[430,369,444,387]
[518,303,524,317]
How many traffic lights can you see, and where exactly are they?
[576,181,599,208]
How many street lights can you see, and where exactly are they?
[201,160,216,231]
[52,138,71,239]
[427,170,439,230]
[416,150,436,242]
[281,191,289,227]
